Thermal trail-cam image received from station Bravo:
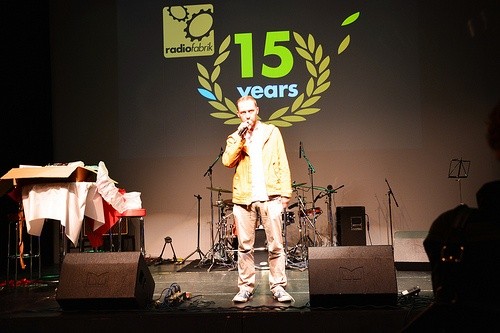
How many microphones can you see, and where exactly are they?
[239,120,252,136]
[288,203,298,209]
[299,142,301,158]
[314,192,321,202]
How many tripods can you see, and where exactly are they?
[180,152,238,273]
[291,187,315,262]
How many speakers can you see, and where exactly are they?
[308,245,398,310]
[336,206,366,246]
[55,251,155,311]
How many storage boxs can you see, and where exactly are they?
[0,161,99,187]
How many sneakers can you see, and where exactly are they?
[273,289,296,302]
[232,290,254,302]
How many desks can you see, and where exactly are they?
[10,183,126,282]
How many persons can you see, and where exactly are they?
[423,100,500,333]
[221,95,292,304]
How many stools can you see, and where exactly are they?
[111,209,146,253]
